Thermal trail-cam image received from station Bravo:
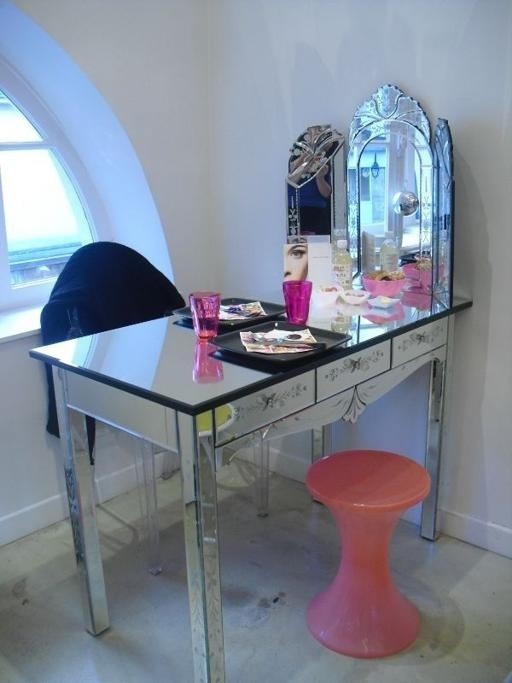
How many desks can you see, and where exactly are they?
[29,299,472,683]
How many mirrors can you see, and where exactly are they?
[347,85,455,301]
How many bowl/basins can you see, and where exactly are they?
[362,272,407,297]
[401,262,444,288]
[339,290,370,305]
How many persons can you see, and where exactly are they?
[283,244,307,282]
[287,128,339,235]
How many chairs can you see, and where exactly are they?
[40,241,269,578]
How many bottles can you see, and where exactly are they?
[379,231,399,272]
[331,240,353,292]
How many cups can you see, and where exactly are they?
[194,342,223,384]
[188,290,220,342]
[282,280,313,325]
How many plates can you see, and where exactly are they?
[367,295,401,309]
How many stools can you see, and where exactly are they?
[305,450,431,660]
